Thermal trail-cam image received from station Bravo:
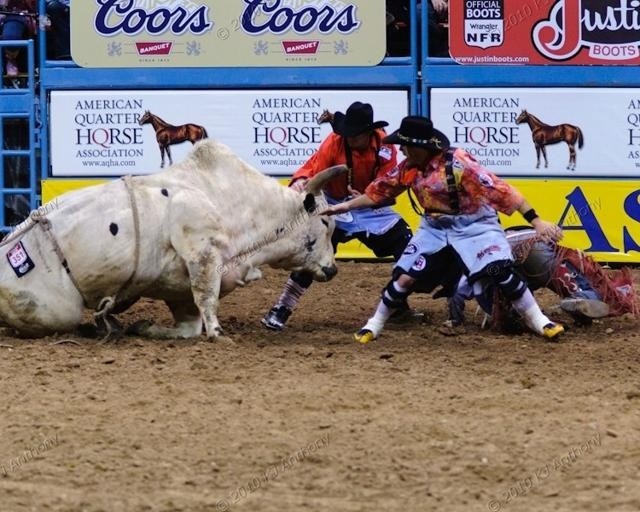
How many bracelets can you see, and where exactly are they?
[523,208,538,225]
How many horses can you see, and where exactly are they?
[139,108,209,168]
[513,108,585,171]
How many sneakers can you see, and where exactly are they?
[439,321,466,335]
[353,328,374,343]
[544,322,564,339]
[560,296,610,319]
[260,305,292,330]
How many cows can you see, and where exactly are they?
[0,134,349,342]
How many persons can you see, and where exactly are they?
[437,217,634,336]
[387,1,449,58]
[258,101,431,329]
[0,0,69,90]
[312,112,565,348]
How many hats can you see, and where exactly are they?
[382,115,450,149]
[333,102,387,137]
